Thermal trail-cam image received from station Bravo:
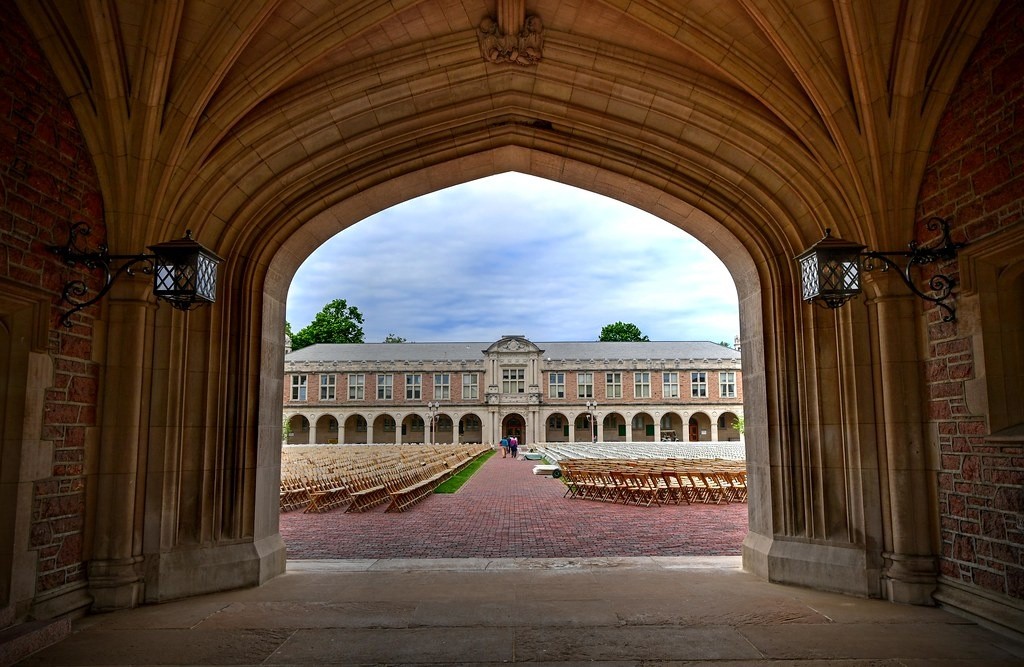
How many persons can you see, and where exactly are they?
[510,436,518,458]
[500,435,508,458]
[505,435,518,454]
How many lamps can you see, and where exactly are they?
[43,220,225,328]
[794,217,967,324]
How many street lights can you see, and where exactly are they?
[585,401,602,443]
[427,401,440,444]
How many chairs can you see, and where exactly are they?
[518,441,748,508]
[280,442,496,514]
